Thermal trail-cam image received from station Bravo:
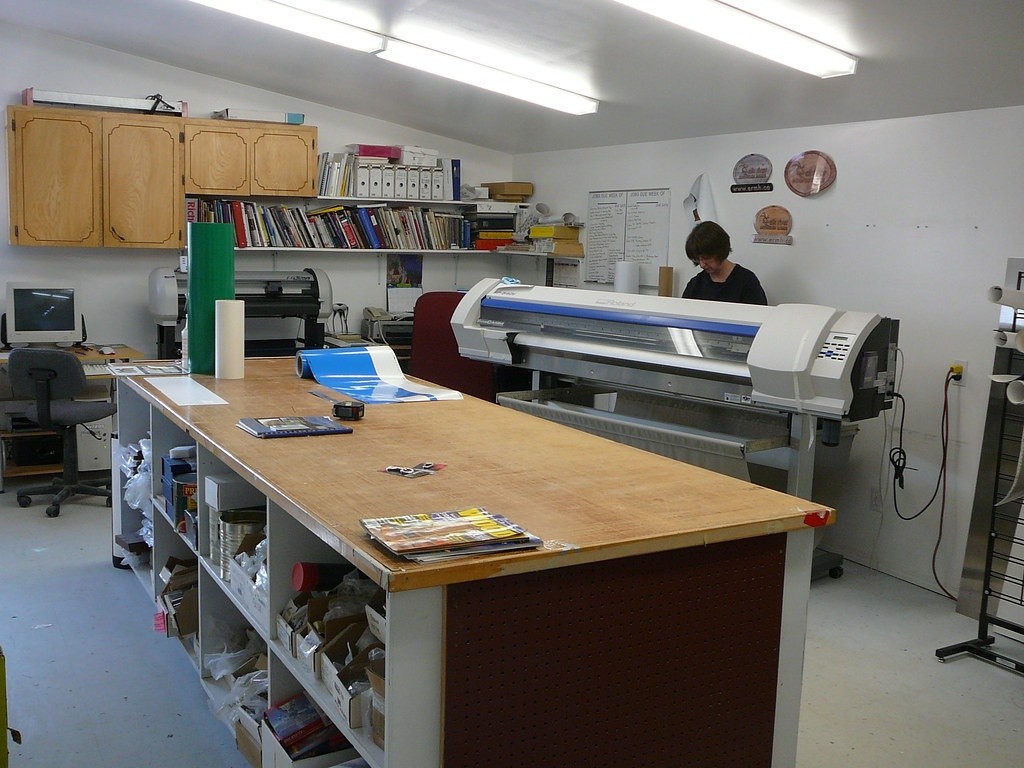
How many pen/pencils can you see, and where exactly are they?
[75,351,87,355]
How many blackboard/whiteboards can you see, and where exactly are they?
[584,188,670,286]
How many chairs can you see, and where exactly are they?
[9,347,119,518]
[412,289,497,403]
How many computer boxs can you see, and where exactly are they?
[74,384,113,471]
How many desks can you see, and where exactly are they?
[0,343,146,495]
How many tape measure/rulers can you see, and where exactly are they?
[332,401,365,420]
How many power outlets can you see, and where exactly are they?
[869,486,884,512]
[950,359,969,387]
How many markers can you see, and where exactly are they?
[584,281,606,283]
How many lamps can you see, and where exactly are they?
[375,36,600,115]
[610,0,859,80]
[188,0,385,53]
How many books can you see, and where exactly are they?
[235,416,353,438]
[314,152,389,196]
[358,508,544,564]
[198,200,473,249]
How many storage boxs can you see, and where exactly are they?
[210,108,305,126]
[346,144,462,202]
[474,187,490,199]
[205,473,268,512]
[162,456,197,486]
[164,499,174,522]
[481,181,533,195]
[158,555,198,591]
[221,533,387,768]
[491,194,523,203]
[154,585,199,639]
[160,477,174,505]
[114,532,145,553]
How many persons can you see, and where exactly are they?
[682,221,768,306]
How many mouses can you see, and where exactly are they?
[99,348,115,355]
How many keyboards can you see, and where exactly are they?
[82,364,110,375]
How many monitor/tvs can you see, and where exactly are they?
[6,281,83,351]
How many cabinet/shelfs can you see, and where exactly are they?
[109,358,834,768]
[6,105,185,250]
[232,195,584,271]
[182,118,320,198]
[364,312,413,360]
